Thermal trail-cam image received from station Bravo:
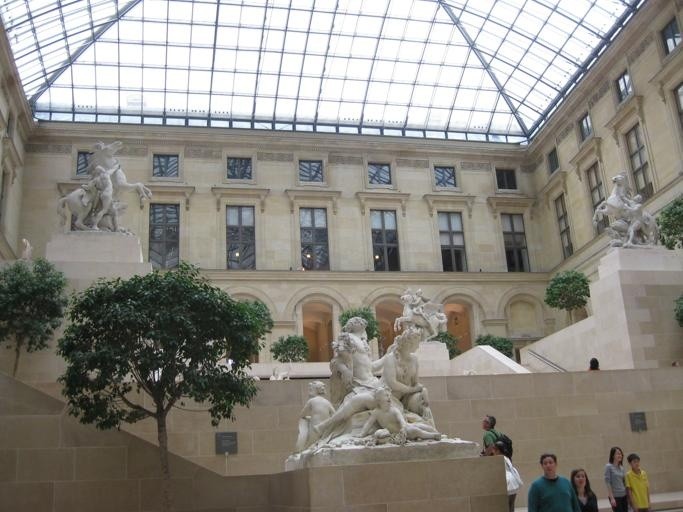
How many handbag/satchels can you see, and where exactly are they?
[505,465,522,496]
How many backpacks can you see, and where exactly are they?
[484,430,513,462]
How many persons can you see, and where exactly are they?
[624,453,652,512]
[492,441,524,512]
[671,360,680,367]
[479,415,503,457]
[619,192,644,249]
[87,163,123,233]
[588,357,600,370]
[527,453,582,512]
[604,446,629,512]
[570,468,599,512]
[299,287,448,446]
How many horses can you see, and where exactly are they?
[592,174,660,248]
[393,294,448,342]
[56,140,151,231]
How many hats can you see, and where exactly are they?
[494,441,506,452]
